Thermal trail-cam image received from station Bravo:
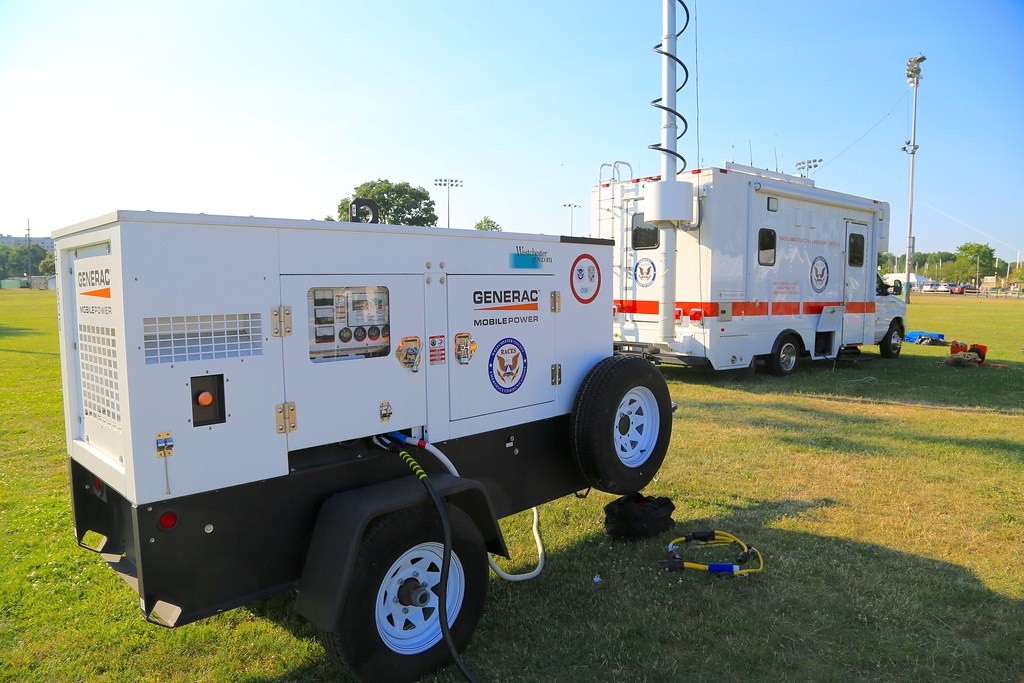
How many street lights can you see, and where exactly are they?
[795,158,823,180]
[433,178,463,228]
[563,202,581,237]
[900,51,929,304]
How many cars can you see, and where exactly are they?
[911,281,982,294]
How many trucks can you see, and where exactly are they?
[589,161,907,376]
